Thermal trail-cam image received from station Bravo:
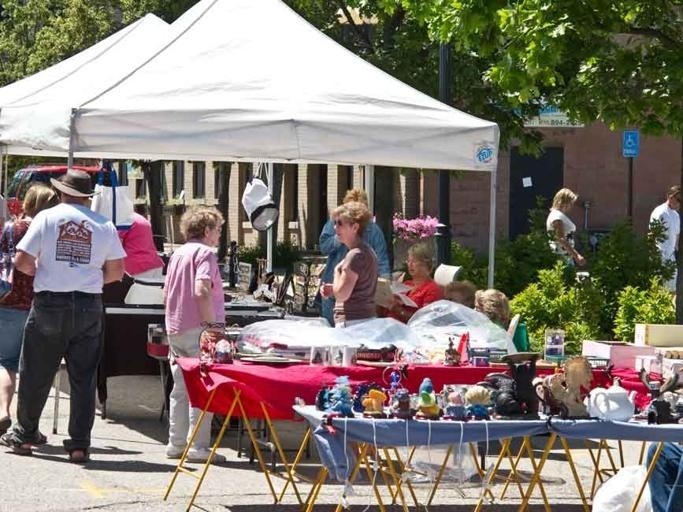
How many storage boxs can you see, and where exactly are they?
[580,338,655,369]
[634,324,683,346]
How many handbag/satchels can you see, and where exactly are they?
[242,163,280,233]
[1,222,17,305]
[92,164,135,230]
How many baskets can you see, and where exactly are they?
[544,354,610,368]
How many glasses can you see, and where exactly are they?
[209,226,222,232]
[334,220,342,226]
[674,196,681,203]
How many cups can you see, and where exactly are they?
[310,346,326,367]
[328,345,348,366]
[342,347,358,367]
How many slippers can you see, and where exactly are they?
[0,416,11,438]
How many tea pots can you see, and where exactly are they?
[593,376,637,424]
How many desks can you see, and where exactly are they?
[102,306,286,375]
[292,404,683,512]
[163,355,671,511]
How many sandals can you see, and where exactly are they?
[68,447,90,462]
[1,433,33,455]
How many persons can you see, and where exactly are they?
[1,188,21,231]
[646,441,682,511]
[318,189,511,330]
[162,206,227,463]
[1,185,62,434]
[1,169,127,463]
[648,185,682,292]
[547,188,586,267]
[115,213,166,305]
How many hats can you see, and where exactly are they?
[50,169,102,198]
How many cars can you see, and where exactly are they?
[0,166,105,218]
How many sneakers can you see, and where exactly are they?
[14,422,48,445]
[187,447,227,464]
[167,443,186,458]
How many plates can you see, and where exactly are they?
[240,356,302,363]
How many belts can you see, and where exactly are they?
[39,291,94,299]
